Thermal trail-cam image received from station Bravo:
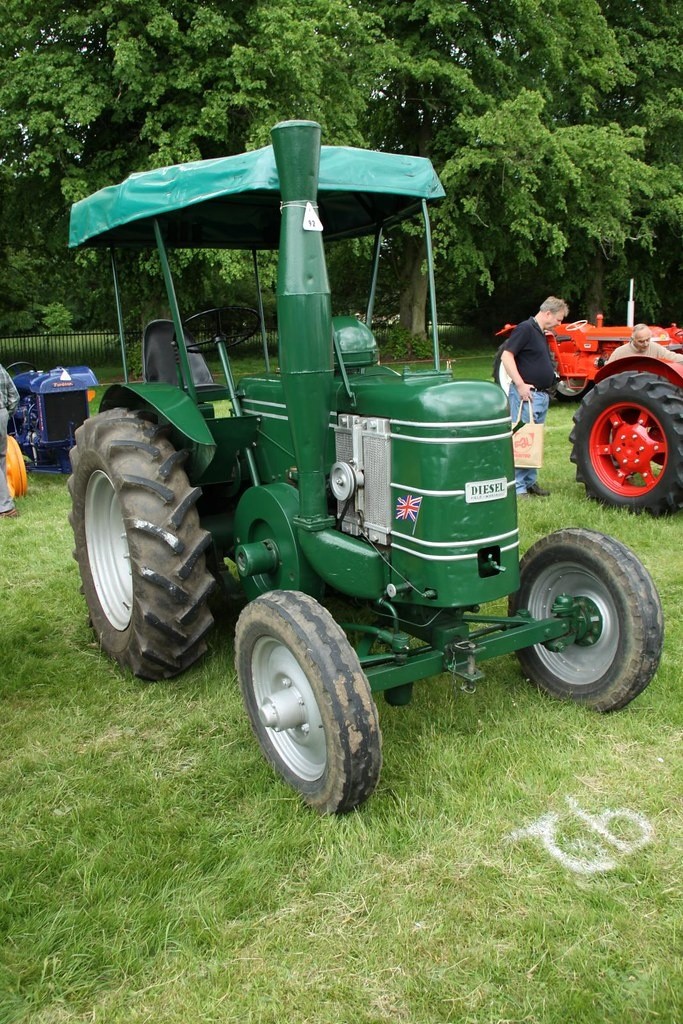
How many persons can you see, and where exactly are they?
[0,363,20,516]
[608,323,683,363]
[500,296,569,497]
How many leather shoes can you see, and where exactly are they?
[518,483,550,499]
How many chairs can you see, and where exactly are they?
[142,319,231,402]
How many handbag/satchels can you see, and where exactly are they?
[510,397,545,469]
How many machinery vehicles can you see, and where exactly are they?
[569,330,683,519]
[62,110,669,815]
[490,278,683,403]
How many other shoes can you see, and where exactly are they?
[0,509,19,518]
